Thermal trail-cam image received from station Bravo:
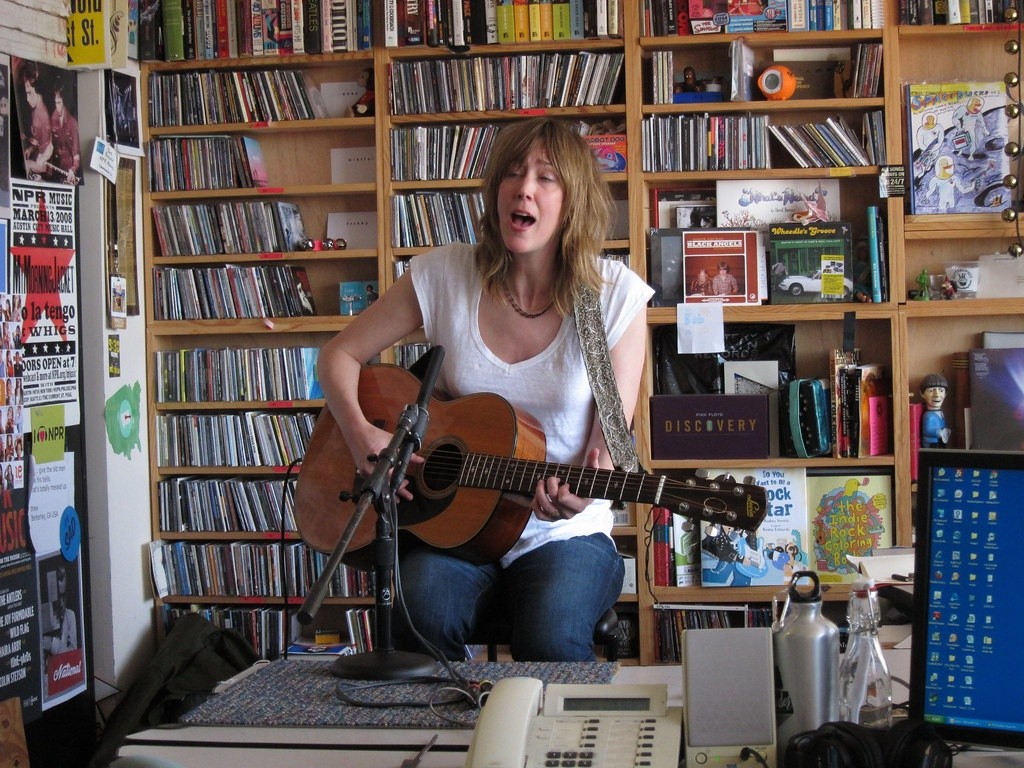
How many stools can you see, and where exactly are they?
[461,603,624,663]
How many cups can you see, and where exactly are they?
[941,260,980,300]
[926,273,946,301]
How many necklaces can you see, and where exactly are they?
[502,283,553,318]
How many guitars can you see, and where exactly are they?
[294,362,770,572]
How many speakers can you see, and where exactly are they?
[681,629,777,768]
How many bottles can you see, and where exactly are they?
[839,578,895,727]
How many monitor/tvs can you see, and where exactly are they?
[909,448,1024,750]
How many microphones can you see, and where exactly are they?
[391,346,446,494]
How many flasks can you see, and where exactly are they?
[769,571,841,758]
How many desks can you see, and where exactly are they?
[116,652,686,764]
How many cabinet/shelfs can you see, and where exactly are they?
[0,0,1023,709]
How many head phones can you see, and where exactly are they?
[784,720,953,768]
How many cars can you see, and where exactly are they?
[780,269,854,299]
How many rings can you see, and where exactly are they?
[551,510,559,517]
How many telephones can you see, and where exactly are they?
[465,676,685,768]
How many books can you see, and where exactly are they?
[641,42,887,174]
[651,605,773,663]
[867,206,888,302]
[388,52,630,370]
[908,81,1012,217]
[830,349,889,458]
[655,469,914,587]
[151,73,377,667]
[131,0,1024,60]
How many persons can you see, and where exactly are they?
[317,125,655,662]
[916,269,929,300]
[0,293,22,490]
[50,78,81,185]
[693,269,713,296]
[941,278,955,299]
[919,374,952,450]
[42,567,78,656]
[366,285,379,306]
[713,260,739,295]
[19,63,54,175]
